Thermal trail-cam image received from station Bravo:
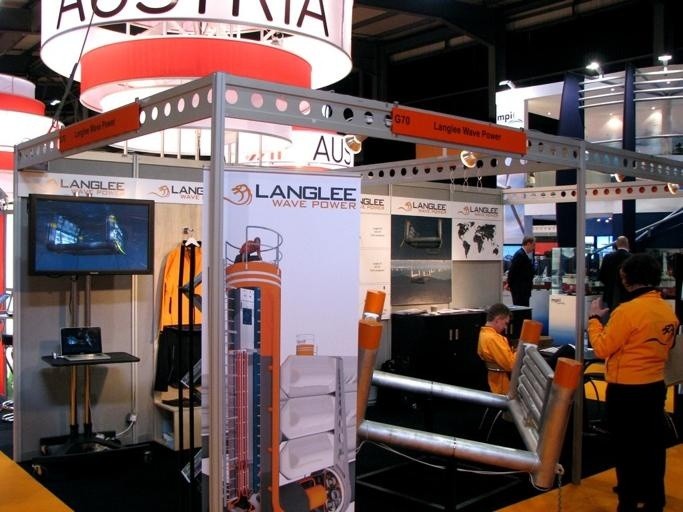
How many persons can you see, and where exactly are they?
[233,237,263,262]
[595,235,634,317]
[476,302,576,423]
[503,236,537,307]
[587,252,681,511]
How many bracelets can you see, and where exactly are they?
[587,315,602,322]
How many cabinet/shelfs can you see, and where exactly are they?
[154,388,205,451]
[391,304,533,419]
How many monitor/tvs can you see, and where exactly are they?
[28,194,154,277]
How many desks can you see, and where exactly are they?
[41,353,140,456]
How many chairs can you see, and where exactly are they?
[464,325,512,442]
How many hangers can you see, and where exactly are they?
[183,229,199,248]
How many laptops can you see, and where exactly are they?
[62,328,111,361]
